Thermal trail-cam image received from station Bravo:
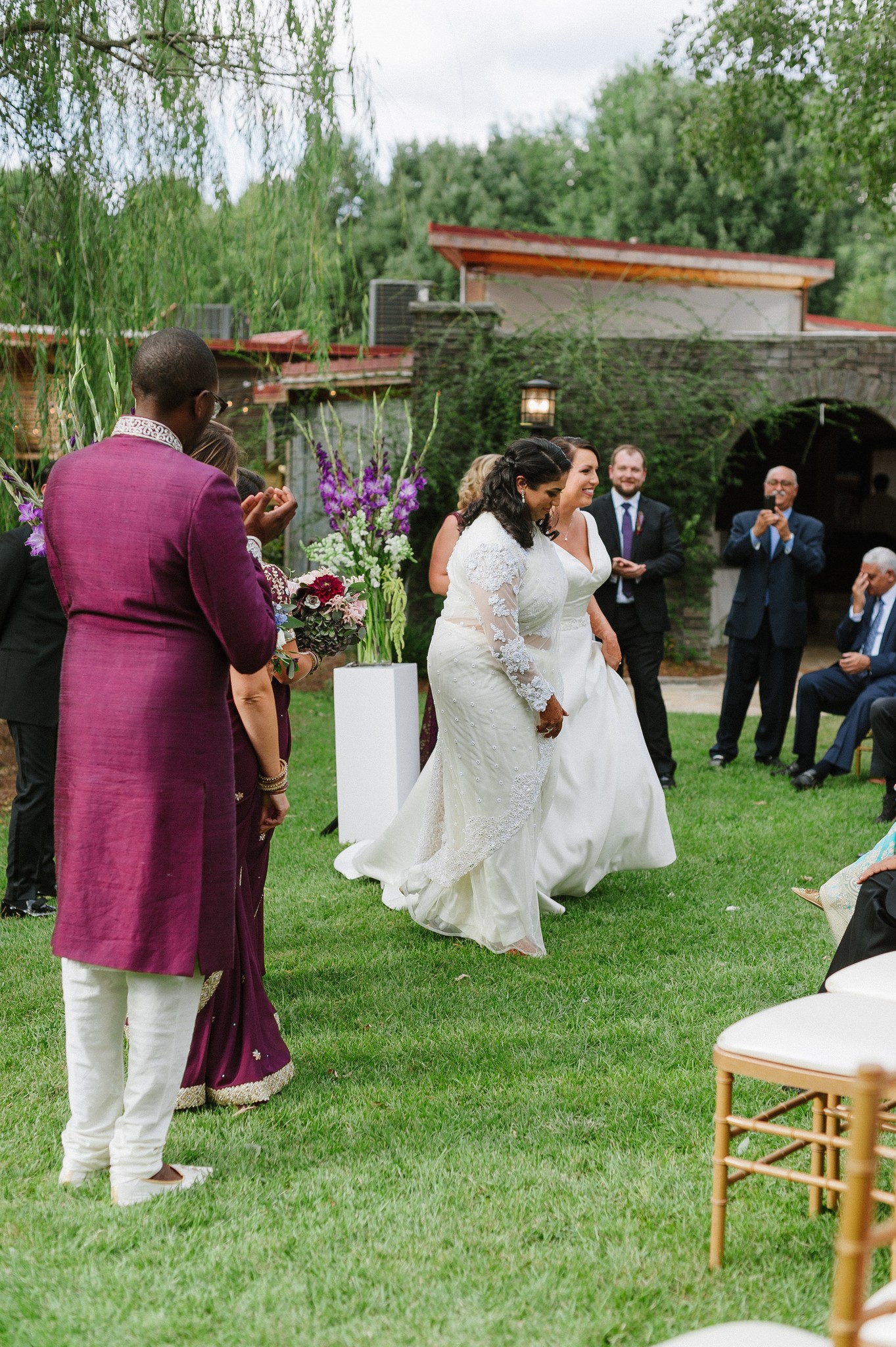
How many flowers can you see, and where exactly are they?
[287,563,364,663]
[294,394,443,660]
[0,455,49,568]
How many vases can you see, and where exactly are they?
[320,658,427,853]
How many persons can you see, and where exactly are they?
[709,465,826,769]
[869,695,896,822]
[581,445,686,786]
[0,461,67,917]
[817,856,896,994]
[334,437,677,957]
[41,327,301,1207]
[790,821,896,946]
[771,547,896,790]
[236,467,323,760]
[173,421,296,1111]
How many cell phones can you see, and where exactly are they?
[764,495,776,514]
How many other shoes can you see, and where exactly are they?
[792,887,824,910]
[707,753,728,767]
[110,1164,212,1207]
[763,757,784,770]
[57,1168,105,1188]
[659,774,677,791]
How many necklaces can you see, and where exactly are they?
[558,522,572,541]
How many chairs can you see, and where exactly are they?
[821,940,895,1002]
[708,983,896,1345]
[843,705,877,792]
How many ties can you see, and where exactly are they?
[860,600,885,677]
[620,503,634,600]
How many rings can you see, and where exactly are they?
[619,662,621,665]
[546,727,553,731]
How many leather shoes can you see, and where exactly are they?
[0,898,57,919]
[875,792,896,823]
[789,769,824,790]
[770,760,810,777]
[36,884,58,898]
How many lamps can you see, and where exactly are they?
[515,369,563,431]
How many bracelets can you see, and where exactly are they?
[300,648,323,675]
[257,758,288,794]
